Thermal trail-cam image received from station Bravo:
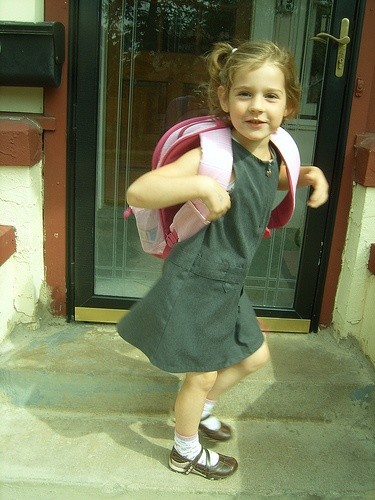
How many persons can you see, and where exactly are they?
[112,34,329,479]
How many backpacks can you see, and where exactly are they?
[129,115,302,256]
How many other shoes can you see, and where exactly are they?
[200,416,232,442]
[167,445,239,481]
